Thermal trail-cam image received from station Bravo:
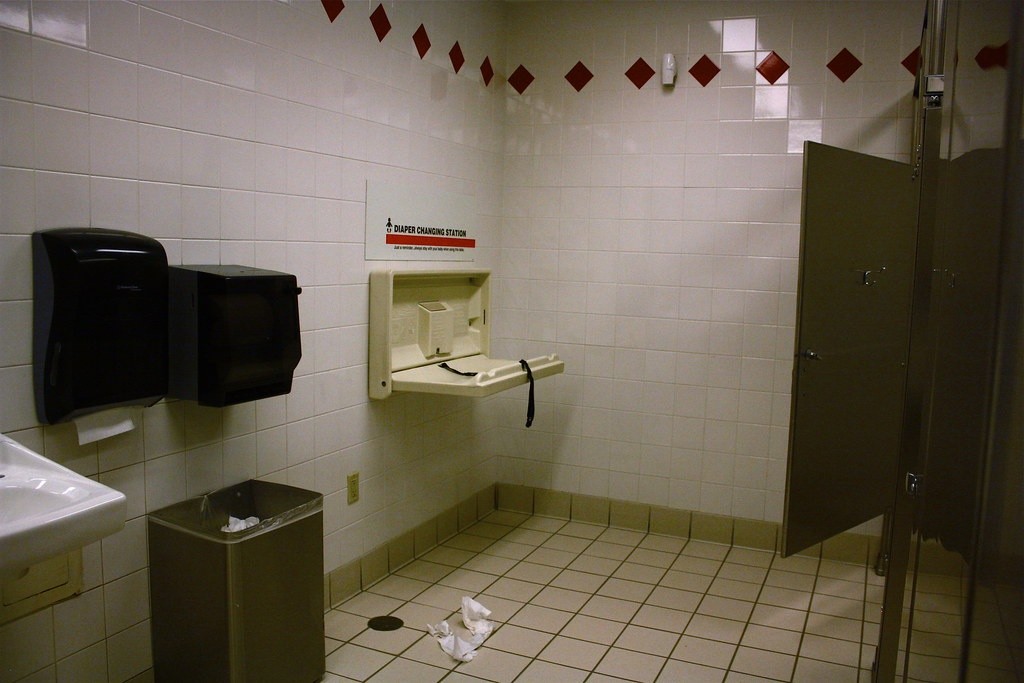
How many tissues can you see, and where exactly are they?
[31,227,170,446]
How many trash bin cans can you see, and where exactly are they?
[144,477,326,683]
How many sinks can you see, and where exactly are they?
[0,433,126,581]
